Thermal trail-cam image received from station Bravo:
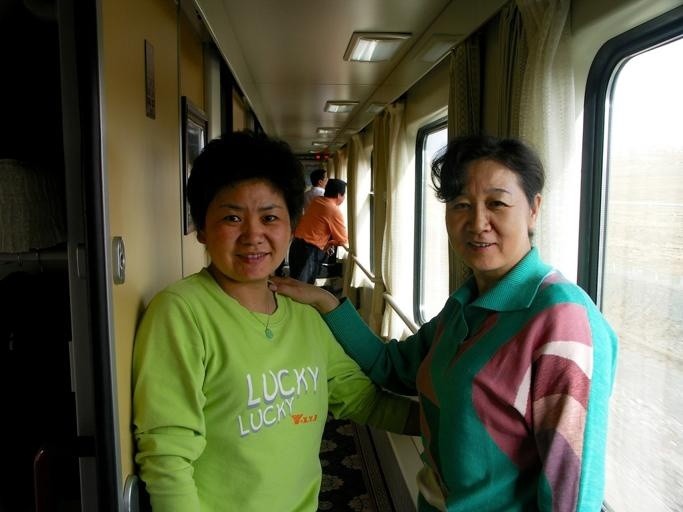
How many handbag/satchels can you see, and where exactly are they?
[314,263,343,294]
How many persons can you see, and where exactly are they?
[266,131,620,511]
[124,127,421,512]
[301,168,329,214]
[286,178,348,288]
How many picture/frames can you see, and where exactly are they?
[182,95,210,237]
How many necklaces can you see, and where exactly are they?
[219,280,279,341]
